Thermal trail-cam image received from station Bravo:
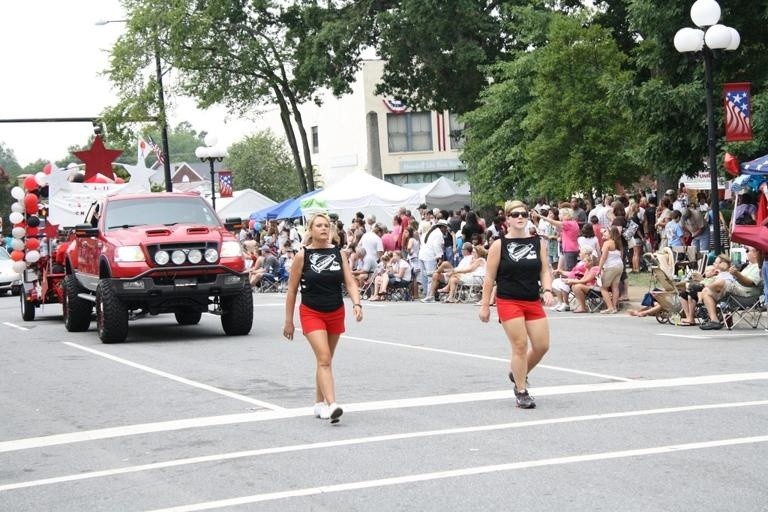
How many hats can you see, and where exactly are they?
[258,245,270,252]
[436,219,448,227]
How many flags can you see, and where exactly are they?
[150,134,163,164]
[137,134,153,166]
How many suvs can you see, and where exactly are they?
[62,191,254,341]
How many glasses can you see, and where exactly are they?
[507,211,529,218]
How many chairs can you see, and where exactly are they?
[255,235,767,335]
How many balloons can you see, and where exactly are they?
[8,163,78,273]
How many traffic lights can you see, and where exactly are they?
[92,118,106,144]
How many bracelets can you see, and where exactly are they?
[542,287,551,295]
[353,303,363,309]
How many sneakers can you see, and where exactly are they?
[328,403,343,423]
[437,289,449,293]
[509,371,536,409]
[421,296,435,303]
[600,307,617,314]
[699,322,722,330]
[444,297,455,303]
[315,403,330,419]
[551,303,589,313]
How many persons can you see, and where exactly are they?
[478,199,558,409]
[282,212,364,423]
[238,183,768,330]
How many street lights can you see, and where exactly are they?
[194,132,228,211]
[674,0,744,258]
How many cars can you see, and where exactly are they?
[0,244,21,297]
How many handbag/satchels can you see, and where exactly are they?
[621,220,640,241]
[641,287,663,307]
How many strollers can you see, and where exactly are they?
[353,264,384,301]
[642,245,721,325]
